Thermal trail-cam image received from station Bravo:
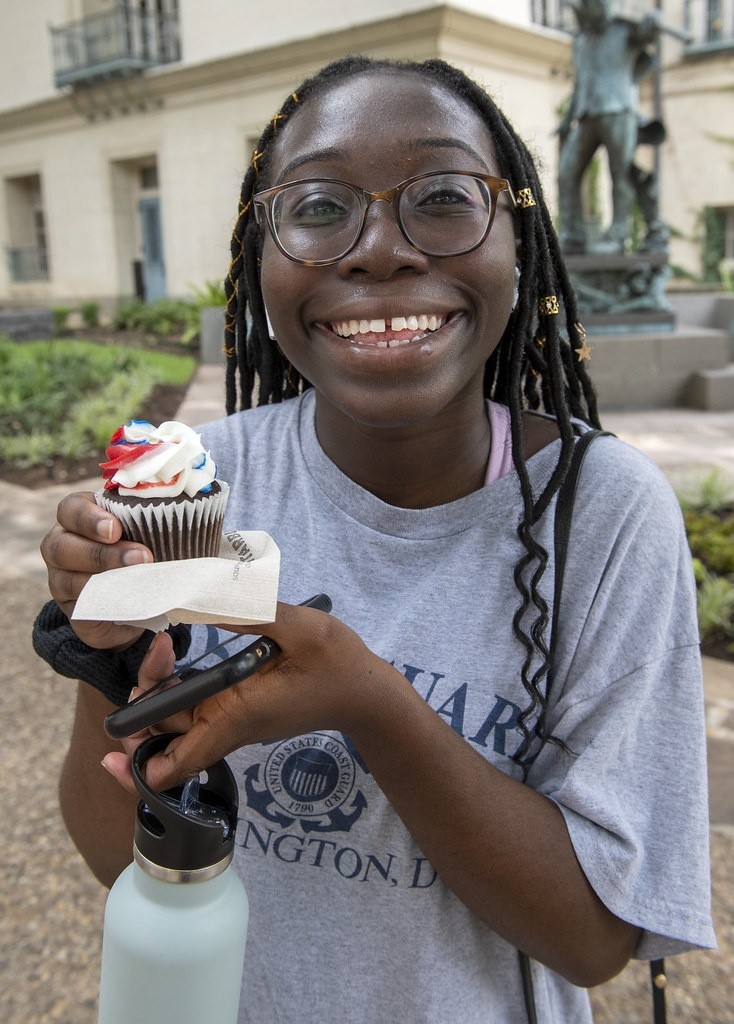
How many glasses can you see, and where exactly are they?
[252,168,520,267]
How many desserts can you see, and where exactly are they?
[95,417,229,562]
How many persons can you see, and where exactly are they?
[38,55,717,1024]
[554,0,661,254]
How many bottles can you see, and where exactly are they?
[99,731,249,1024]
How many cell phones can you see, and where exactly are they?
[103,594,333,741]
